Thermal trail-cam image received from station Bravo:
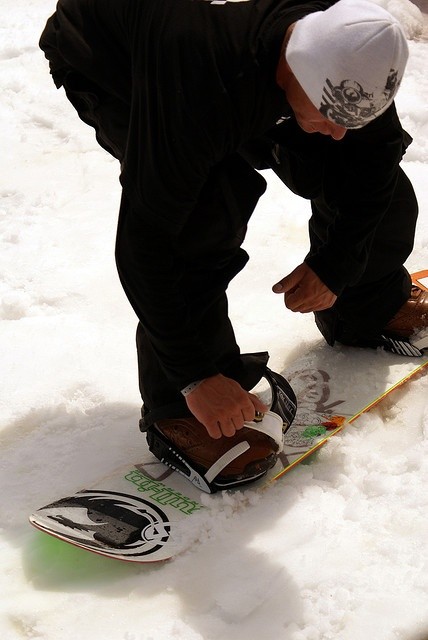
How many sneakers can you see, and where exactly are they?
[140,405,278,487]
[313,284,428,353]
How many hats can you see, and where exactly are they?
[285,2,408,130]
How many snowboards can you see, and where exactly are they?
[27,270,428,562]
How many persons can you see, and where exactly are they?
[38,0,427,491]
